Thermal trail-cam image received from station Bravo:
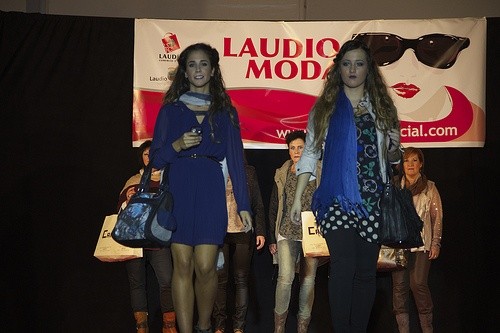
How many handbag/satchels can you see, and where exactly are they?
[94,215,143,262]
[301,211,330,257]
[111,184,174,248]
[381,183,425,249]
[377,246,408,272]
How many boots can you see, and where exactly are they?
[163,313,177,333]
[134,312,149,333]
[296,315,311,333]
[274,310,288,333]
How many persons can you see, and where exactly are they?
[391,147,443,333]
[214,152,266,333]
[116,141,175,333]
[148,43,253,333]
[290,39,401,333]
[267,129,319,333]
